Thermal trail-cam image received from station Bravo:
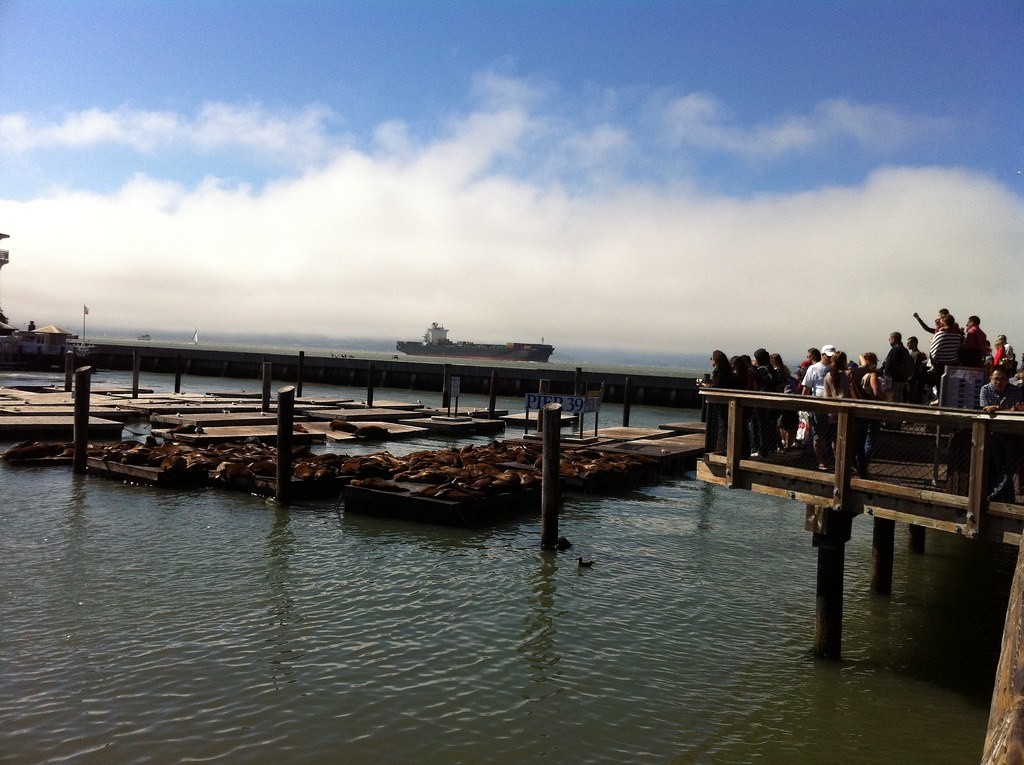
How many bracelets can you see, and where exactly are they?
[997,405,999,409]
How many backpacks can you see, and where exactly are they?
[848,368,878,400]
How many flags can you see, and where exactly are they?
[84,307,88,314]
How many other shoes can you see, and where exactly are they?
[714,442,804,459]
[857,466,869,479]
[926,393,938,405]
[819,463,827,470]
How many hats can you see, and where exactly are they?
[821,345,836,356]
[753,349,771,365]
[864,353,877,363]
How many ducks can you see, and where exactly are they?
[574,557,597,567]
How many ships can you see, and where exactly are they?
[396,321,555,364]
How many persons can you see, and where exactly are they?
[697,308,1024,502]
[801,344,836,470]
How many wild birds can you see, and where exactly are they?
[467,410,474,416]
[485,407,488,410]
[417,400,421,403]
[474,408,478,412]
[660,448,670,454]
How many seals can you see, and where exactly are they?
[329,418,394,435]
[57,435,656,497]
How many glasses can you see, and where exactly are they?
[807,356,809,358]
[709,357,715,361]
[989,376,1008,382]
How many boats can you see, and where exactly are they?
[137,334,151,341]
[0,323,98,368]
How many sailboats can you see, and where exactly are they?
[188,329,199,345]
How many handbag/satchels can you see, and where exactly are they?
[796,410,809,441]
